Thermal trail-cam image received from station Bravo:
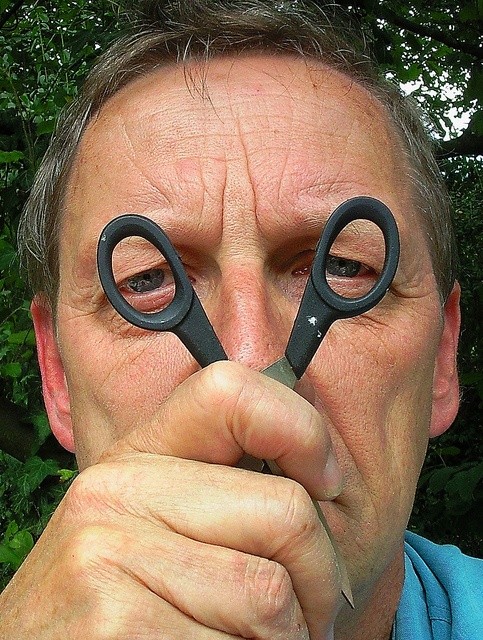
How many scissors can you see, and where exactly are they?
[97,196,399,610]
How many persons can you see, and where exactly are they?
[1,0,481,635]
